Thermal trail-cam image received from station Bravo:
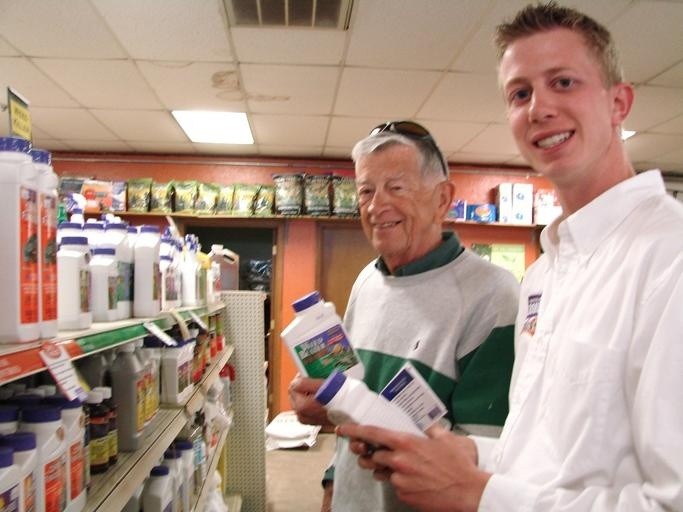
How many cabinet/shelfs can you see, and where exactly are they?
[0,303,247,512]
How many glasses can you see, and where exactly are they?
[369,122,447,178]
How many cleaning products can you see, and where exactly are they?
[1,313,218,509]
[314,370,431,448]
[1,134,219,339]
[280,292,365,388]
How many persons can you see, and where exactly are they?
[286,121,523,512]
[335,0,683,512]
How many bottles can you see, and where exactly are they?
[282,294,427,456]
[0,136,237,512]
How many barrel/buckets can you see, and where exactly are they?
[208,243,239,290]
